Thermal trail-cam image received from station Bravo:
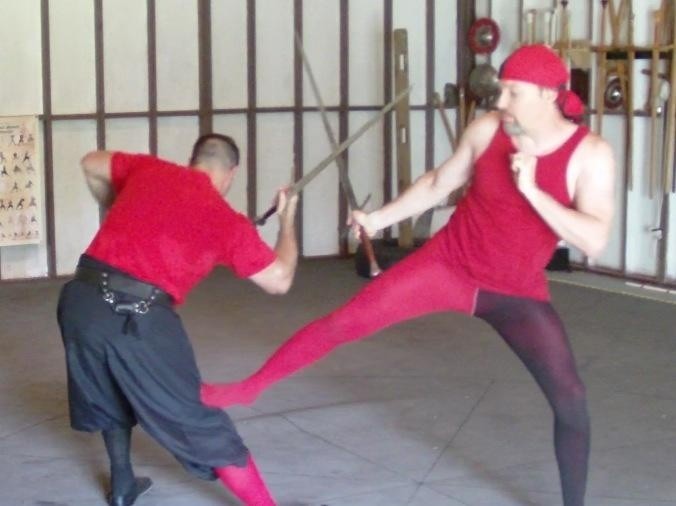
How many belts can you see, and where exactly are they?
[75,264,172,308]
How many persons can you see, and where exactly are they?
[200,43,616,506]
[55,130,299,506]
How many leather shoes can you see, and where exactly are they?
[108,477,152,506]
